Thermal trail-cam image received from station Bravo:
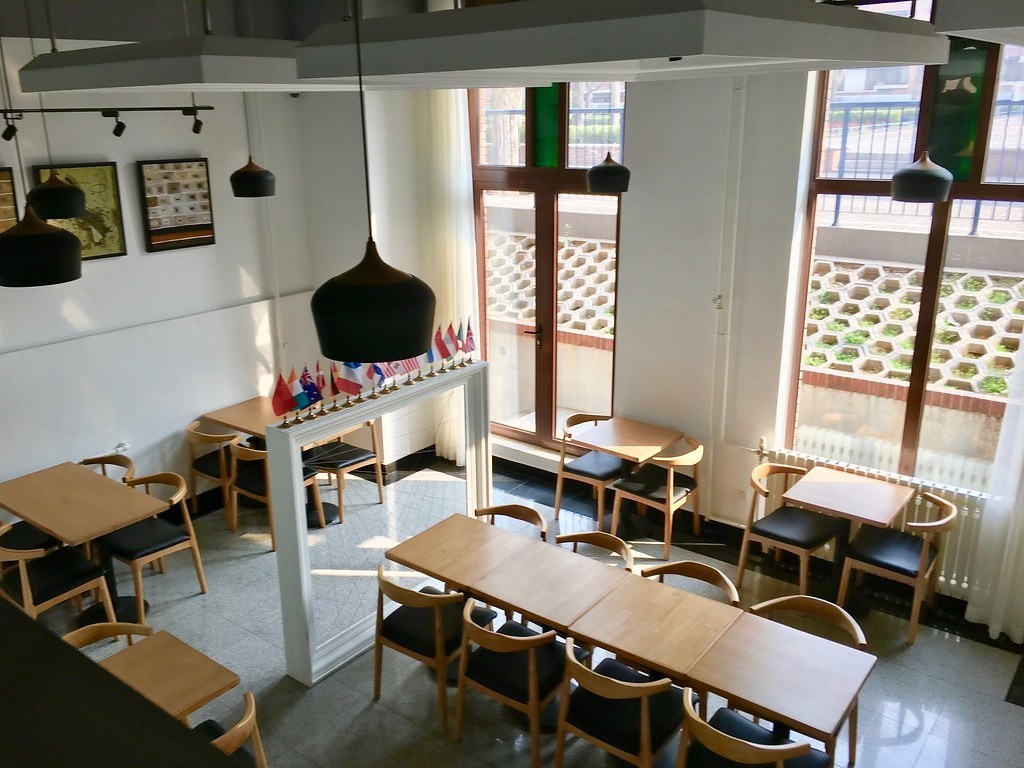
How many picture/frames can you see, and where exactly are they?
[0,167,20,235]
[32,162,127,261]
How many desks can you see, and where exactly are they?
[571,416,684,540]
[0,462,170,627]
[202,396,338,530]
[384,514,877,768]
[97,630,240,727]
[781,466,915,622]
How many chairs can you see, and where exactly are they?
[445,505,547,622]
[555,637,700,768]
[610,435,704,561]
[616,560,739,722]
[554,414,642,531]
[375,564,497,734]
[193,693,268,768]
[187,415,384,551]
[734,462,851,600]
[0,454,208,642]
[521,529,634,671]
[676,687,831,768]
[837,492,958,646]
[61,622,153,649]
[728,594,868,764]
[453,598,591,768]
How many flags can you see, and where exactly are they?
[271,317,477,417]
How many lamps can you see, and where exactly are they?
[102,111,125,137]
[137,157,216,253]
[892,65,953,202]
[183,109,202,134]
[587,83,630,192]
[21,1,86,220]
[230,92,275,197]
[310,1,435,363]
[2,113,22,141]
[0,40,81,288]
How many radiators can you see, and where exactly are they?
[761,447,986,601]
